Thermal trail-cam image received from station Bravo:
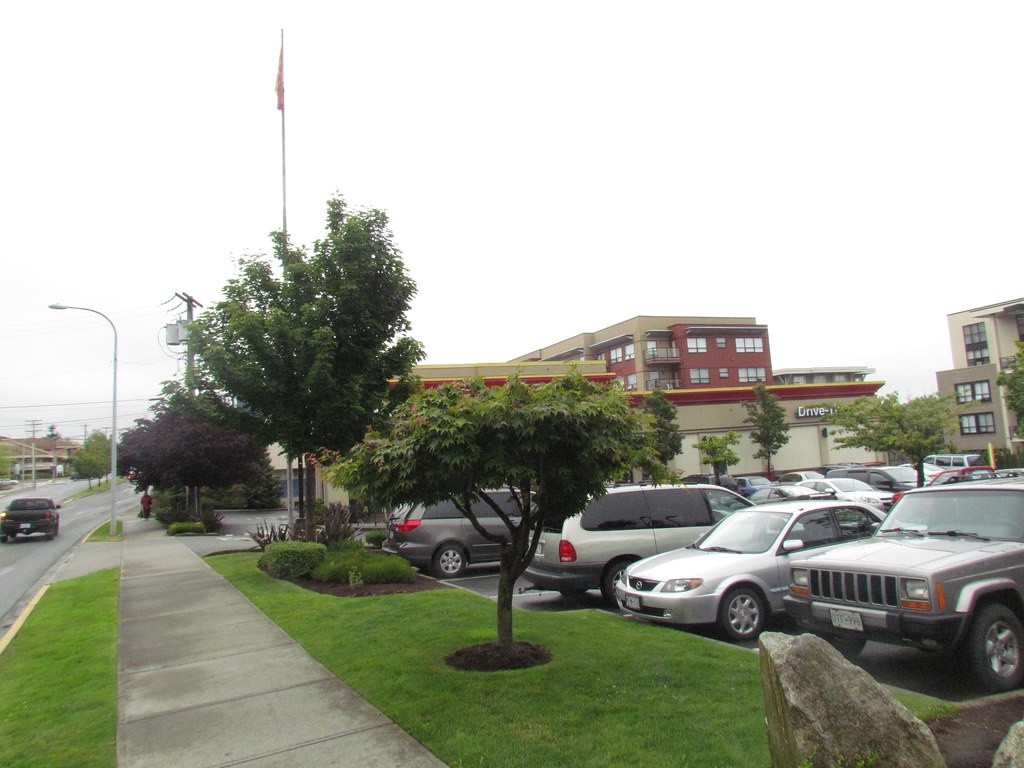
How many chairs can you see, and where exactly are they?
[666,384,673,390]
[765,517,785,544]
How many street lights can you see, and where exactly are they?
[164,318,201,516]
[48,304,118,538]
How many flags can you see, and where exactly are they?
[275,46,284,110]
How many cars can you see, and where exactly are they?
[0,497,62,544]
[614,499,889,642]
[678,453,1024,512]
[0,478,18,486]
[329,522,390,555]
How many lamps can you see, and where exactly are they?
[822,428,828,438]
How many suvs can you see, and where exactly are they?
[380,484,543,579]
[782,473,1023,695]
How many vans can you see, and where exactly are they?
[516,483,758,600]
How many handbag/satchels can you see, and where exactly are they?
[141,511,143,518]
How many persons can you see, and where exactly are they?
[140,491,153,521]
